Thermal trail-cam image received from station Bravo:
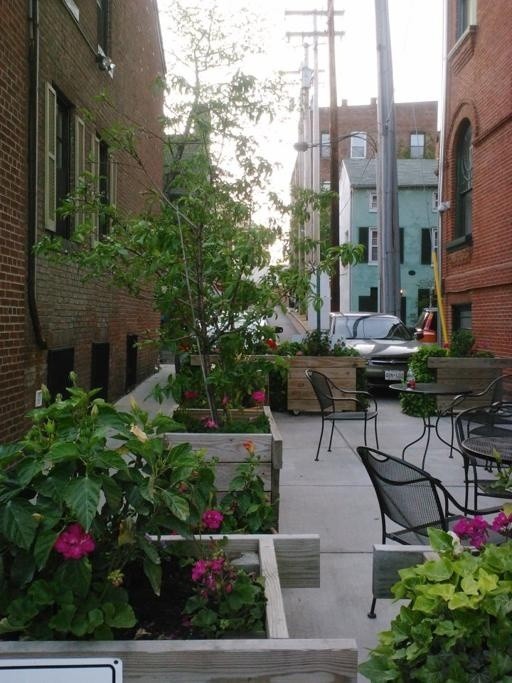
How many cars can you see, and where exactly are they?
[323,310,428,391]
[196,303,284,345]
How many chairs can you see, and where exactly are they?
[450,374,512,519]
[306,367,379,461]
[355,447,512,618]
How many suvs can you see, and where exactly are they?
[409,304,437,343]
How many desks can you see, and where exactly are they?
[389,383,473,471]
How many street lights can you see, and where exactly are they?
[290,128,405,316]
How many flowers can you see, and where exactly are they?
[220,395,231,406]
[201,413,218,430]
[55,524,96,558]
[252,388,268,404]
[183,389,195,398]
[192,553,234,599]
[199,504,225,530]
[267,338,274,350]
[356,503,512,683]
